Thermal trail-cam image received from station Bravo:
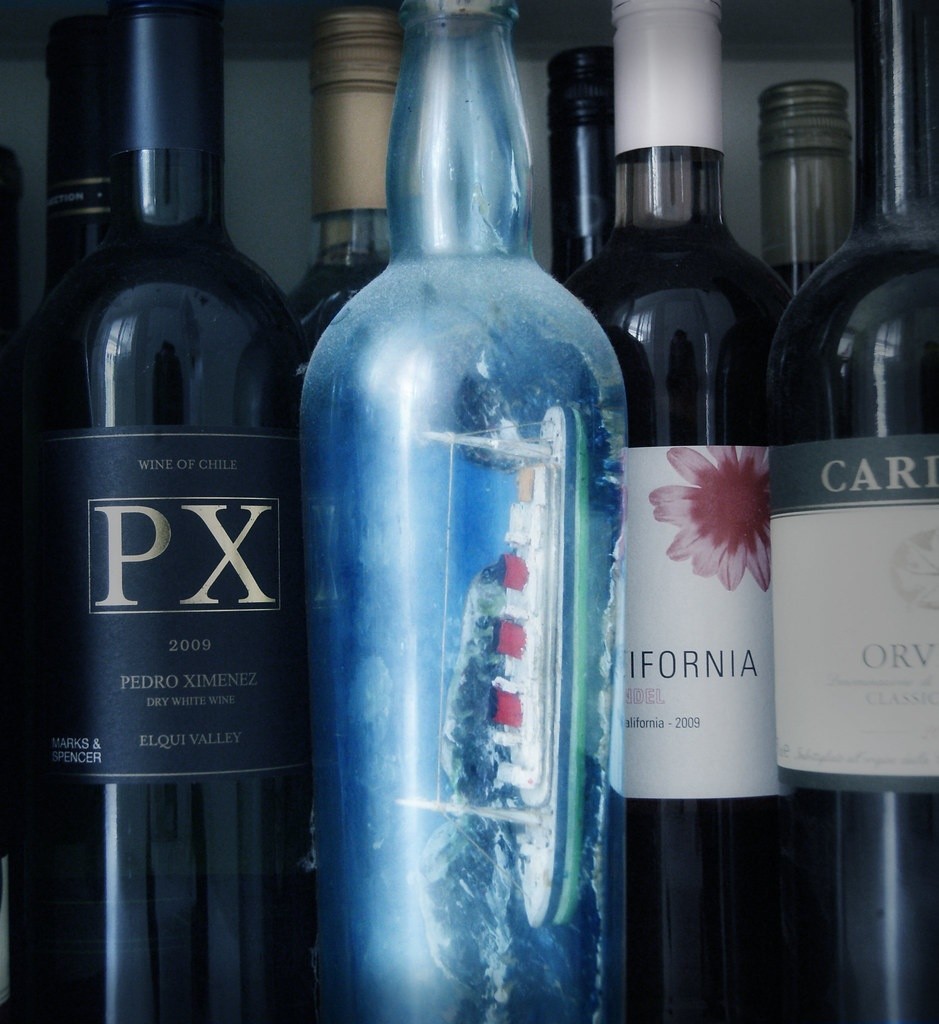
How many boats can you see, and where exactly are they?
[400,403,592,933]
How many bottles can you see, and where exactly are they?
[550,0,798,1024]
[289,0,628,1024]
[0,0,300,1024]
[757,0,939,1024]
